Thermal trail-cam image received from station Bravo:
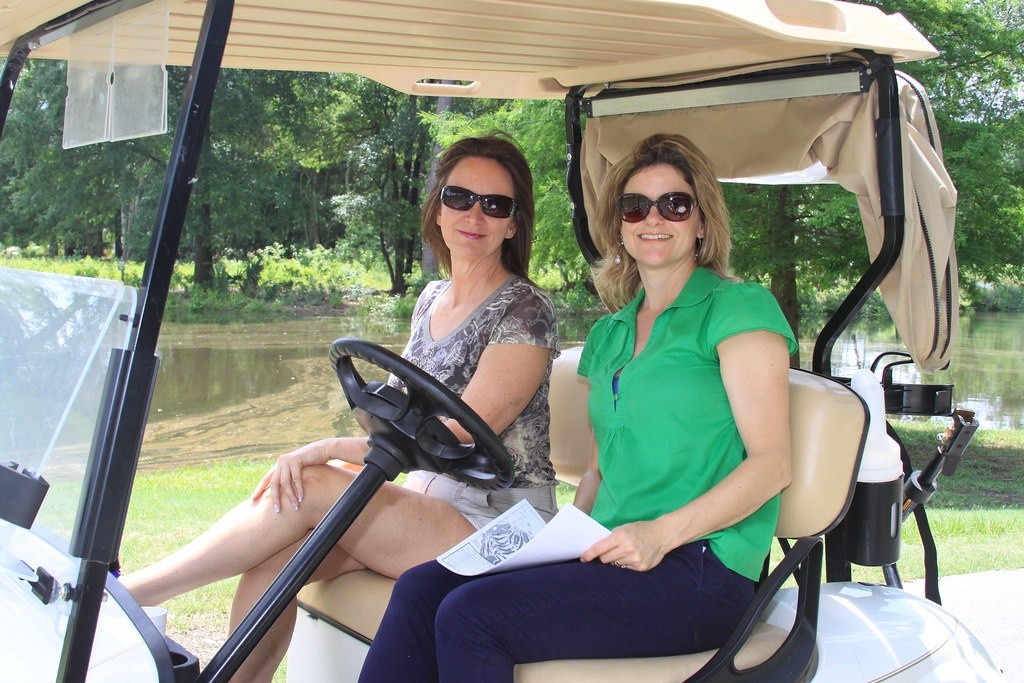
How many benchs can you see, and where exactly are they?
[285,341,873,683]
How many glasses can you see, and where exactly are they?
[440,185,517,218]
[616,192,699,222]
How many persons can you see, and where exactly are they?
[92,129,566,683]
[355,130,801,683]
